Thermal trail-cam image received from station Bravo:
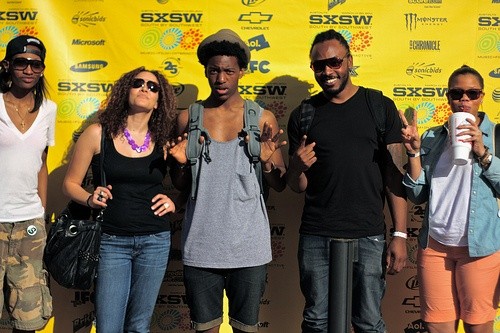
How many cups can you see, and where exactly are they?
[447,111,476,166]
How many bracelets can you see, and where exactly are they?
[263,160,274,173]
[87,194,96,209]
[393,231,407,239]
[406,151,420,158]
[477,146,492,167]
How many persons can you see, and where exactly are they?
[290,28,407,333]
[398,64,500,333]
[169,29,288,333]
[0,35,55,333]
[63,66,193,333]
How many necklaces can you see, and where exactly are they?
[13,94,32,127]
[122,129,150,153]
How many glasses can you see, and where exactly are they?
[9,58,46,73]
[131,78,161,93]
[309,51,350,73]
[447,88,482,100]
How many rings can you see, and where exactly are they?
[163,203,169,209]
[97,196,102,202]
[99,191,103,196]
[266,137,272,141]
[404,124,409,127]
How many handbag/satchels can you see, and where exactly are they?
[42,212,102,290]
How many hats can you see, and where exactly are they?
[197,30,251,68]
[4,35,46,63]
